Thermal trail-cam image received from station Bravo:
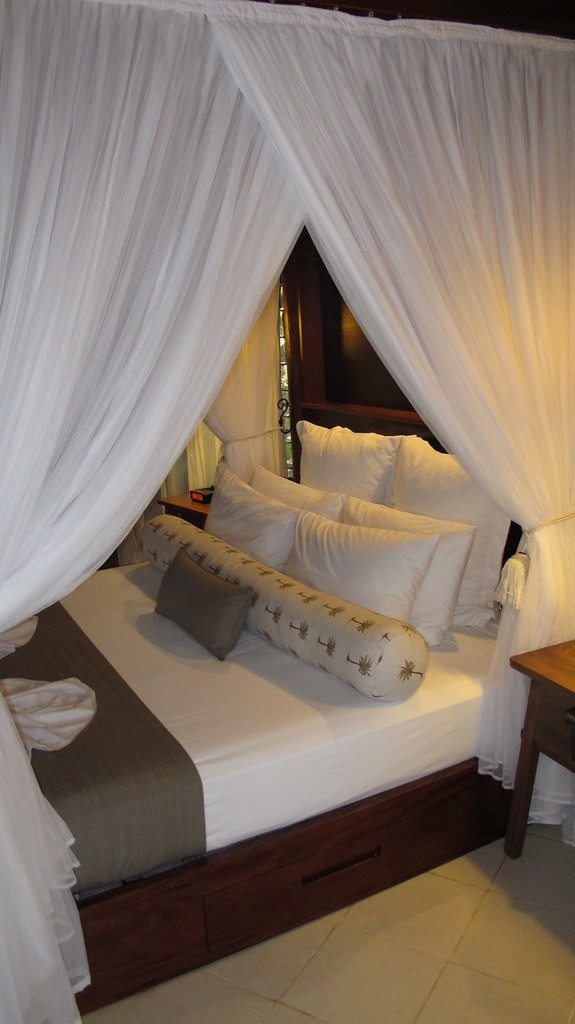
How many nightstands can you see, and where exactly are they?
[505,639,575,860]
[157,494,211,531]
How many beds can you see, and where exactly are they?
[0,401,522,1015]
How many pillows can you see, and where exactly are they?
[141,514,431,703]
[296,420,417,504]
[204,461,301,574]
[155,546,253,661]
[248,464,344,524]
[341,497,476,648]
[284,509,440,623]
[393,437,511,627]
